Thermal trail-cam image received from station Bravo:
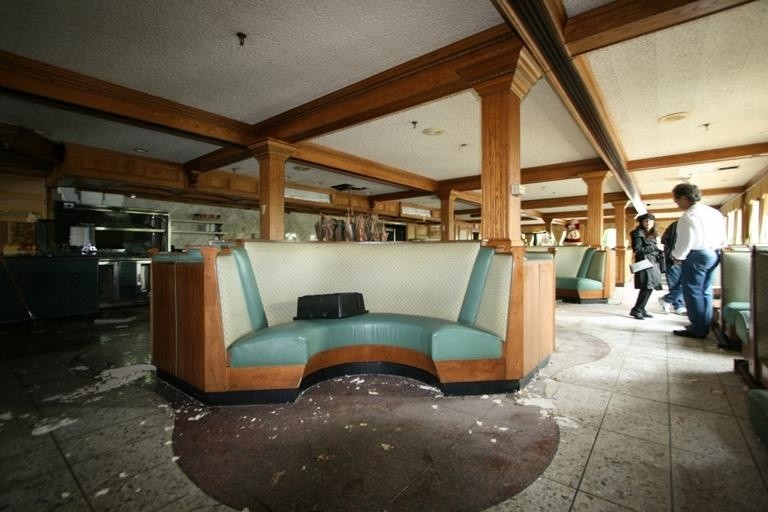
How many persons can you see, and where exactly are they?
[630,213,664,321]
[669,183,726,338]
[660,221,688,315]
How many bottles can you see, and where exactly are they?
[193,213,222,222]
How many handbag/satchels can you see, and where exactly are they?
[629,259,654,274]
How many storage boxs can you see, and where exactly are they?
[293,293,368,319]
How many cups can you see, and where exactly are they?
[203,223,215,232]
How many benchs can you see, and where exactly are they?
[711,246,768,389]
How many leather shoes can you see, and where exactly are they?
[673,325,706,340]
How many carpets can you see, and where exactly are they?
[172,397,560,512]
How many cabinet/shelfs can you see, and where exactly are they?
[55,204,170,308]
[171,219,229,240]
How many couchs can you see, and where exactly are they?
[528,245,611,304]
[201,239,527,405]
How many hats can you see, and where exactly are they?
[638,214,654,221]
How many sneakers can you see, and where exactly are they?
[658,297,671,314]
[675,307,688,315]
[630,307,654,321]
